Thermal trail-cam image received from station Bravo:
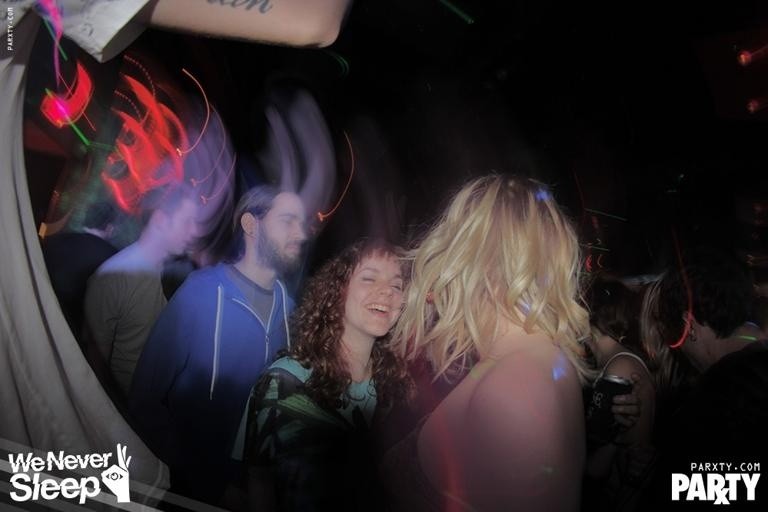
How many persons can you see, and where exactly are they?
[1,0,768,511]
